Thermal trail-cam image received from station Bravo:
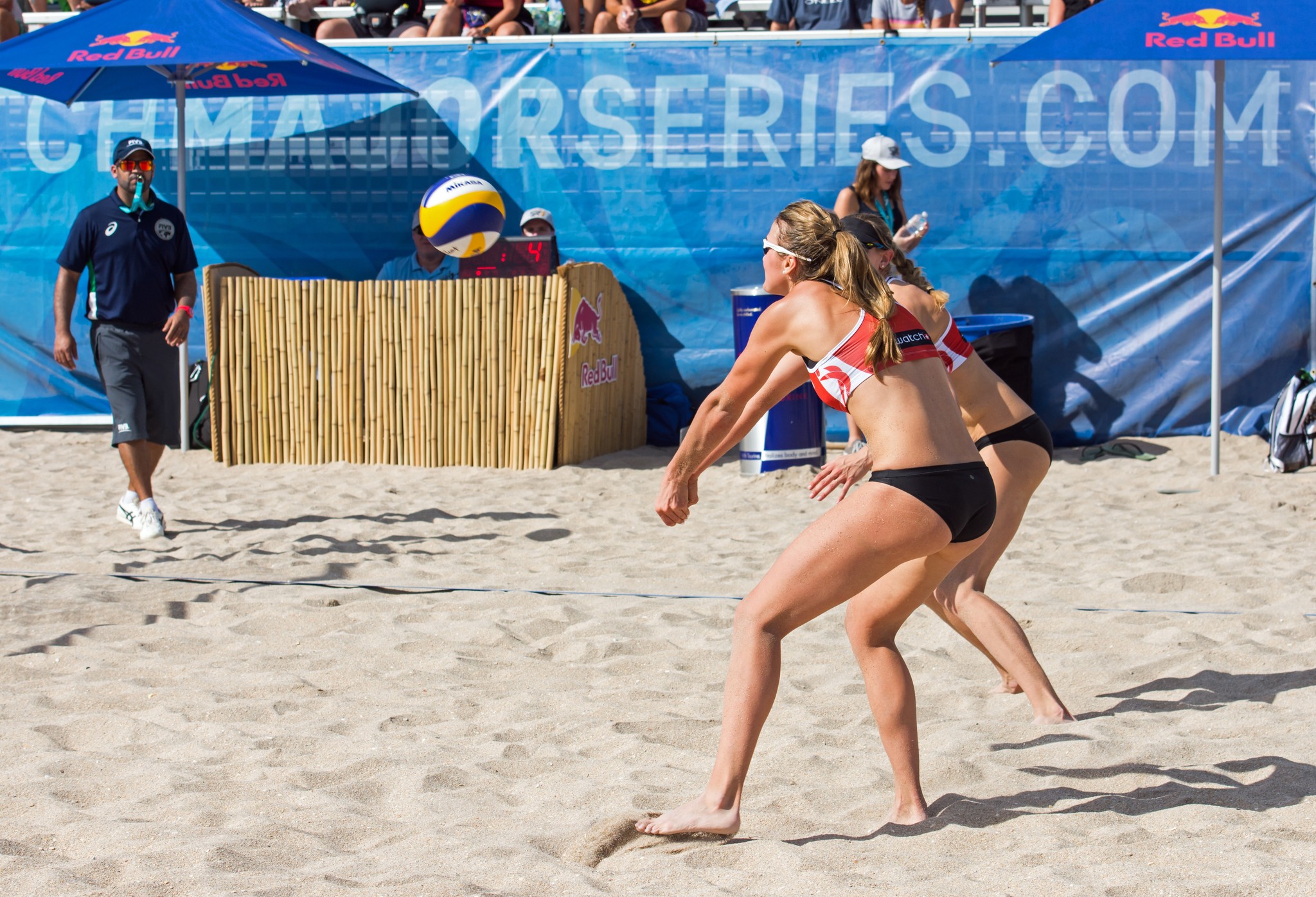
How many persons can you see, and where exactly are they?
[53,137,199,544]
[377,213,460,281]
[523,209,560,273]
[949,0,967,28]
[845,138,929,458]
[0,0,102,41]
[1048,0,1091,29]
[808,213,1075,723]
[240,0,709,41]
[634,201,1000,839]
[871,0,955,30]
[765,0,873,31]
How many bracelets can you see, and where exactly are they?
[635,8,643,21]
[483,24,491,36]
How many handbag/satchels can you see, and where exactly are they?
[186,360,213,448]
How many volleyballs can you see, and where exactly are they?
[419,173,506,259]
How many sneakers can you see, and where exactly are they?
[140,508,166,539]
[116,495,141,528]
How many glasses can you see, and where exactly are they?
[117,160,153,172]
[414,228,424,235]
[859,239,887,250]
[763,238,812,262]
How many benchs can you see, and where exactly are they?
[22,0,1049,32]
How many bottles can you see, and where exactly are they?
[905,211,928,235]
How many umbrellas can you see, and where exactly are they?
[0,0,421,451]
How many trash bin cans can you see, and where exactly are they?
[950,312,1033,443]
[730,284,826,478]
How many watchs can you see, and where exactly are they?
[173,305,195,320]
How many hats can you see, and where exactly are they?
[519,207,554,229]
[838,217,878,243]
[412,208,426,229]
[861,133,912,170]
[113,137,155,166]
